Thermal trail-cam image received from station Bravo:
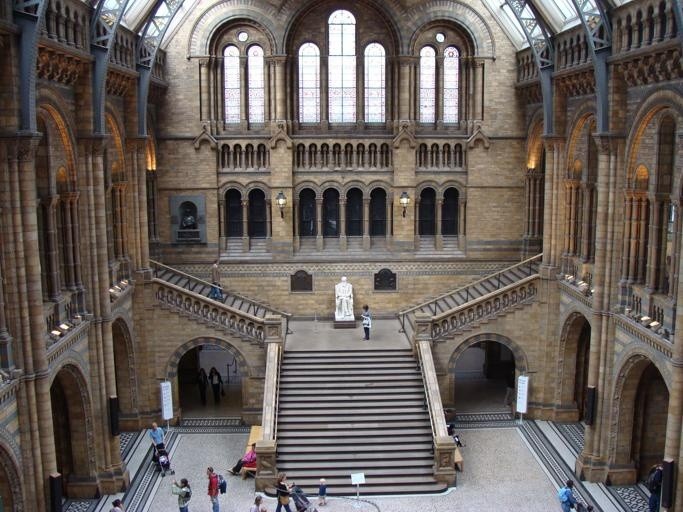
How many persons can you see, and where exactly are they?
[172,479,191,512]
[207,467,219,512]
[211,260,223,300]
[227,443,256,474]
[448,423,463,446]
[195,368,208,405]
[276,472,295,512]
[361,304,371,339]
[336,277,352,319]
[250,496,263,512]
[261,507,266,512]
[150,423,165,452]
[208,367,223,404]
[319,478,328,506]
[109,499,125,512]
[558,481,593,511]
[650,466,662,511]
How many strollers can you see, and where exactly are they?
[153,442,175,477]
[288,485,319,512]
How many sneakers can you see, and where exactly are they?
[227,468,241,476]
[319,503,326,505]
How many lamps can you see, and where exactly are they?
[273,188,291,221]
[397,188,412,222]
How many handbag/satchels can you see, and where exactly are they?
[281,495,291,505]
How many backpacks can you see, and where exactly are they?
[559,486,570,502]
[220,480,226,494]
